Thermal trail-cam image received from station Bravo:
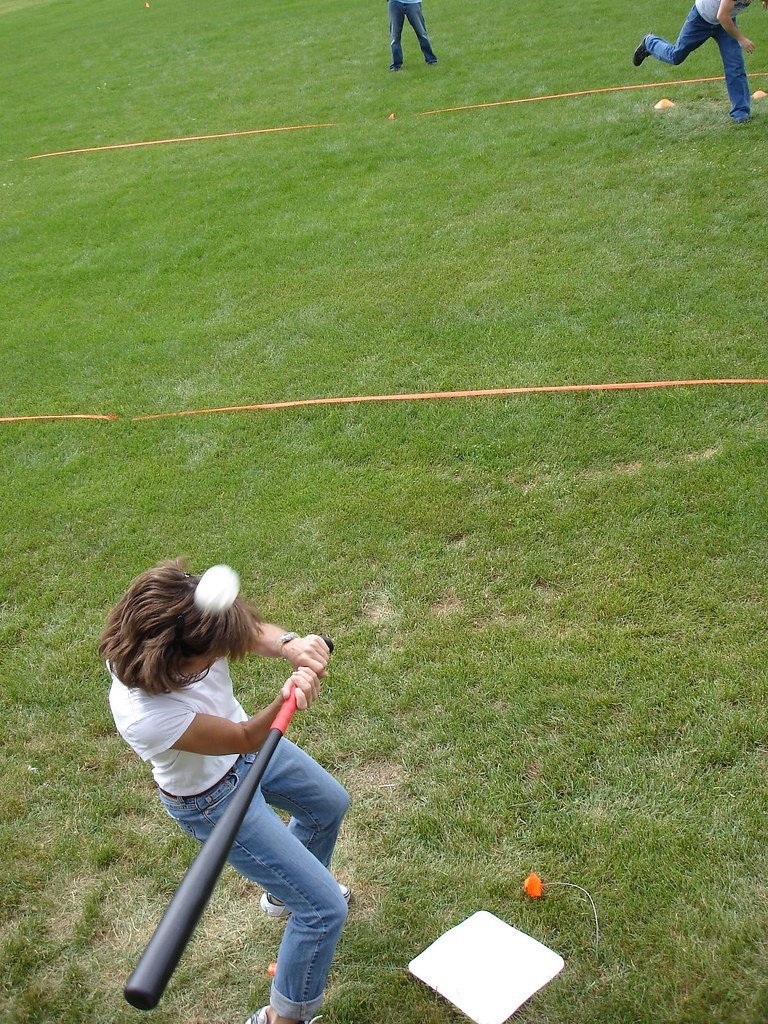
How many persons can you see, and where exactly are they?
[93,556,349,1024]
[632,0,768,123]
[387,0,437,72]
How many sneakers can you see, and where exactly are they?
[260,883,350,917]
[633,35,651,66]
[244,1005,323,1024]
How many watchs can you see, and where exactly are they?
[278,632,300,658]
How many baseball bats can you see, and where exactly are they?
[121,634,337,1012]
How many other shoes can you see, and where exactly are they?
[432,62,437,65]
[390,67,398,71]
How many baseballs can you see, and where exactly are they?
[191,563,243,616]
